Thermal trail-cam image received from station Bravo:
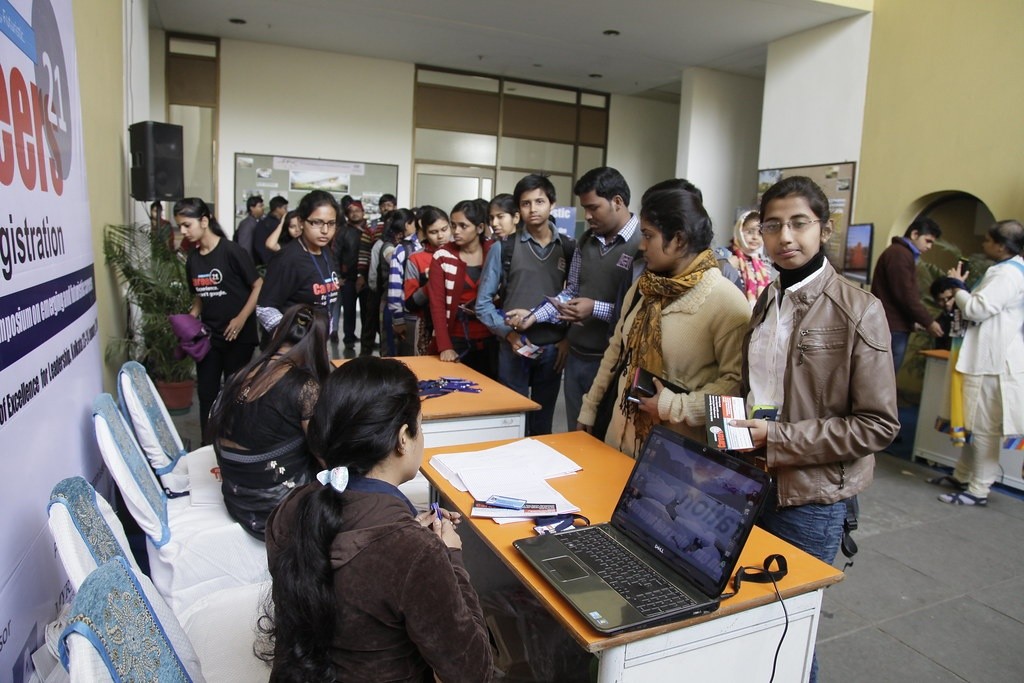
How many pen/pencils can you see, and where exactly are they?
[627,396,642,405]
[431,502,442,520]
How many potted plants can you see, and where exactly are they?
[92,216,196,414]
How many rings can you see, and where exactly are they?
[234,331,237,334]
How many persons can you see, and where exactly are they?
[929,273,955,350]
[174,197,264,447]
[266,210,302,251]
[576,178,752,460]
[739,175,902,683]
[342,169,579,438]
[207,303,333,543]
[149,200,174,260]
[252,196,290,264]
[505,166,645,442]
[724,209,771,309]
[925,219,1024,506]
[251,355,494,683]
[255,191,340,350]
[870,216,944,456]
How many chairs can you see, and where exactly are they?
[47,361,274,683]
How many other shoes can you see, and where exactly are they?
[329,330,338,340]
[342,335,359,343]
[361,340,381,351]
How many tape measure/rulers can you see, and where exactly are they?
[474,501,556,510]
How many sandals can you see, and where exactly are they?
[927,477,969,490]
[939,492,988,506]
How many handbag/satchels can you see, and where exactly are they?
[590,283,644,442]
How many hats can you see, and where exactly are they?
[344,200,365,212]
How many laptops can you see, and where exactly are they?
[512,424,771,637]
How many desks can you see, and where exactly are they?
[420,430,845,683]
[331,356,542,446]
[909,349,1024,488]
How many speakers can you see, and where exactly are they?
[129,120,185,203]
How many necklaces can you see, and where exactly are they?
[267,351,297,368]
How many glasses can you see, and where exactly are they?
[307,218,337,230]
[757,221,818,234]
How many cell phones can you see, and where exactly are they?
[626,382,640,403]
[748,404,779,421]
